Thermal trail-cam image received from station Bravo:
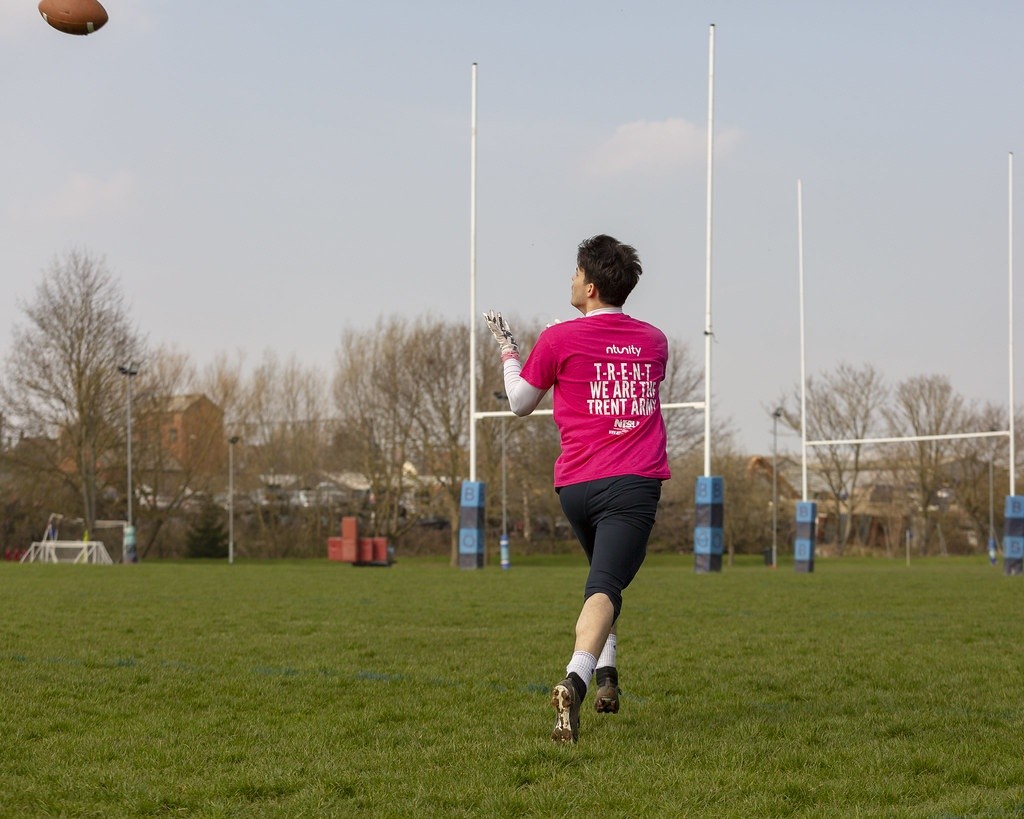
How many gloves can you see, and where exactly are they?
[483,310,519,354]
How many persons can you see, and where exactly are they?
[480,234,669,743]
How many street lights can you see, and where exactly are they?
[771,406,784,566]
[121,359,140,563]
[227,436,240,564]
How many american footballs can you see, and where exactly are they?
[39,0,109,36]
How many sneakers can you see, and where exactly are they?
[594,678,622,714]
[551,678,580,745]
